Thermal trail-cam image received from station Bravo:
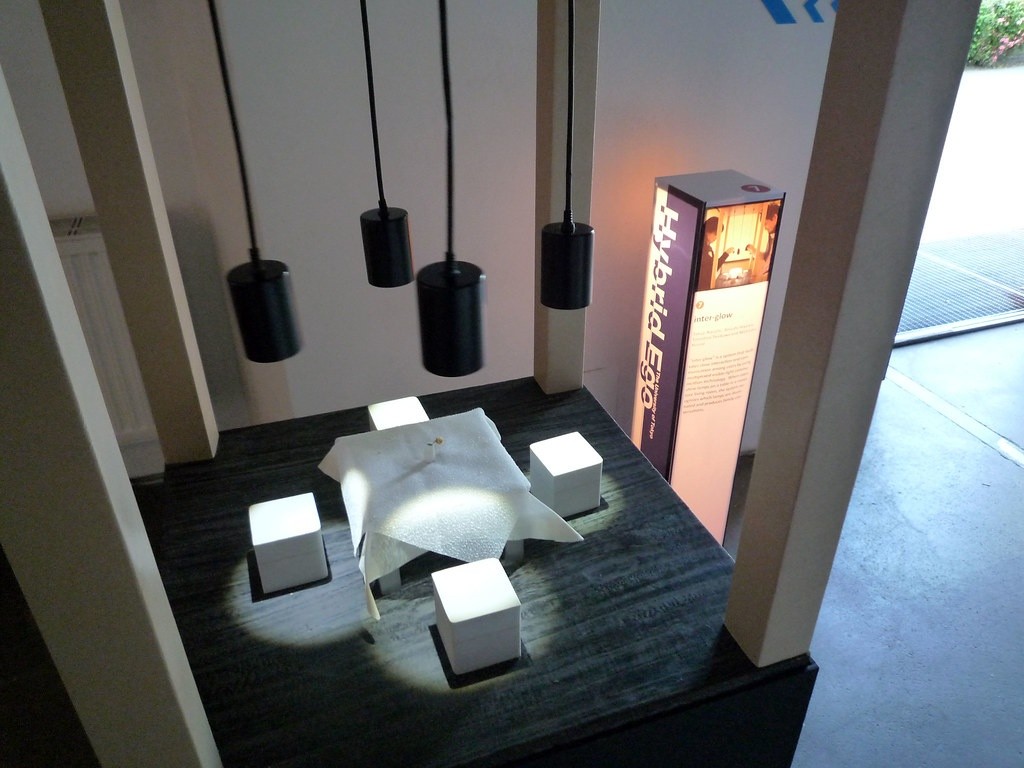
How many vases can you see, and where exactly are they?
[426,443,436,464]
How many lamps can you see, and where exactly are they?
[207,0,304,365]
[415,0,486,379]
[358,0,415,289]
[538,0,597,309]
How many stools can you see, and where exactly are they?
[529,432,603,518]
[428,557,522,675]
[247,492,329,595]
[368,398,431,431]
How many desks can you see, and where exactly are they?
[317,407,584,623]
[0,377,823,768]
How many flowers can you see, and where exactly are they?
[432,436,444,447]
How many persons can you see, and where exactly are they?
[695,201,780,290]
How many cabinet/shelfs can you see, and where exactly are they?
[47,212,161,450]
